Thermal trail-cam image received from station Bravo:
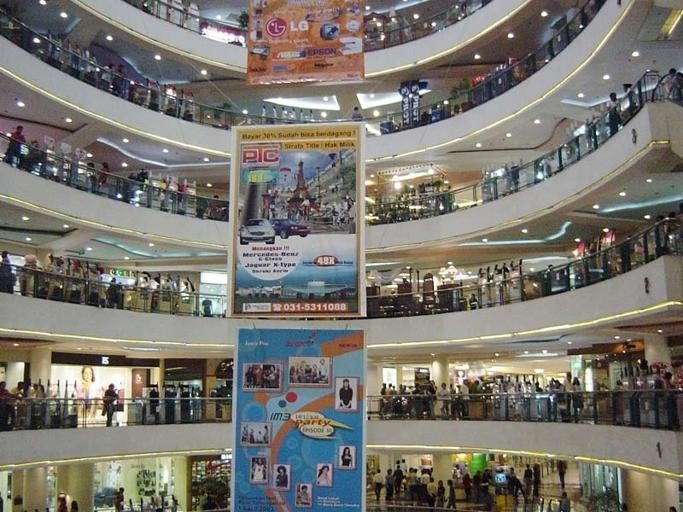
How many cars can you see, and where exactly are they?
[239,218,275,245]
[271,219,310,240]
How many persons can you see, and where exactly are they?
[242,358,332,507]
[341,447,352,466]
[339,379,353,409]
